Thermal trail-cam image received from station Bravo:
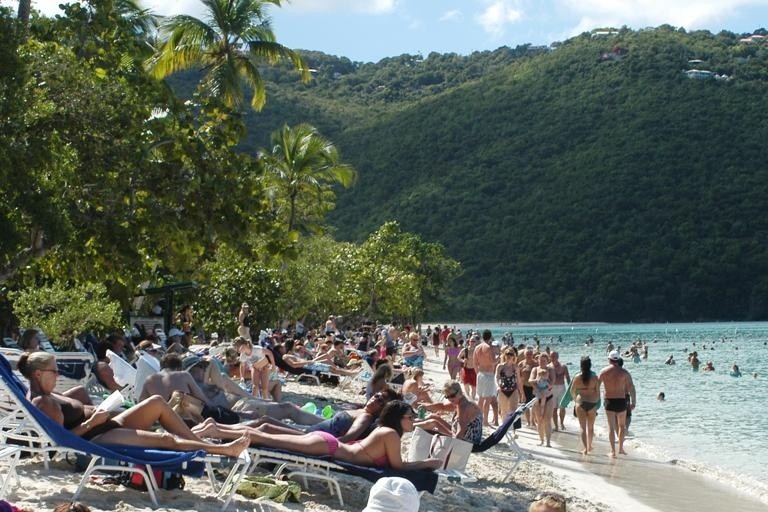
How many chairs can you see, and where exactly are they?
[426,396,540,483]
[0,354,250,510]
[4,336,75,377]
[266,349,337,386]
[211,434,478,508]
[34,325,94,374]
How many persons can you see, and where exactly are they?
[508,332,514,347]
[547,351,570,432]
[366,364,404,402]
[231,337,275,400]
[326,314,336,336]
[426,326,432,336]
[95,333,138,399]
[456,329,461,346]
[613,358,636,438]
[569,355,599,455]
[729,364,742,378]
[180,356,328,426]
[190,398,443,472]
[16,327,44,358]
[495,347,526,440]
[441,326,450,353]
[399,326,412,344]
[472,329,498,426]
[372,319,388,343]
[533,341,540,353]
[356,333,380,367]
[63,329,95,380]
[15,352,251,460]
[501,332,510,347]
[478,341,497,423]
[271,309,315,360]
[532,334,537,341]
[173,305,192,324]
[132,339,162,371]
[518,348,538,429]
[345,327,356,344]
[138,353,258,431]
[411,379,483,447]
[401,368,433,408]
[315,329,333,355]
[527,352,557,447]
[432,327,441,357]
[691,351,701,372]
[164,329,206,356]
[446,329,456,339]
[199,389,405,443]
[665,355,675,364]
[310,343,363,370]
[443,337,460,381]
[180,332,193,349]
[657,391,665,402]
[237,302,252,345]
[333,335,363,371]
[595,350,633,456]
[374,328,398,362]
[682,334,739,353]
[402,331,426,381]
[457,340,465,376]
[222,347,281,403]
[607,336,648,364]
[584,335,593,347]
[704,361,715,371]
[687,353,693,363]
[282,339,364,379]
[457,335,480,400]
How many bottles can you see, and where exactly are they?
[417,400,426,420]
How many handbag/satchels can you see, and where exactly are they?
[166,390,206,422]
[408,426,473,474]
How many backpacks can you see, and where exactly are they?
[103,458,185,490]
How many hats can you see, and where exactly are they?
[182,357,209,372]
[608,350,619,360]
[347,359,357,366]
[169,328,185,336]
[144,343,161,352]
[242,303,249,308]
[469,335,477,341]
[362,476,419,512]
[334,335,349,345]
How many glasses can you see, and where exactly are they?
[445,389,459,399]
[374,392,385,407]
[411,339,419,341]
[401,414,414,420]
[196,364,208,369]
[41,368,58,373]
[504,353,514,356]
